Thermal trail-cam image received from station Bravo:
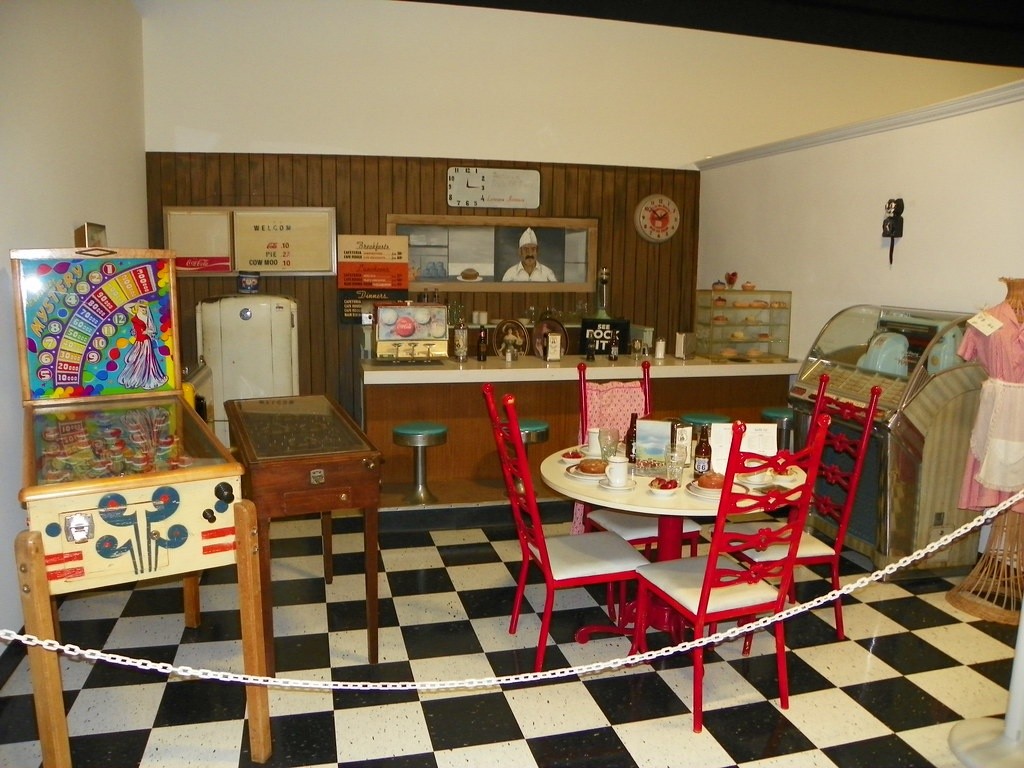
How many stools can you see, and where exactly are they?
[392,422,448,506]
[761,406,794,449]
[681,412,732,440]
[503,419,548,499]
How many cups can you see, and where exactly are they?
[525,299,590,325]
[587,428,601,453]
[746,459,766,482]
[665,444,687,487]
[598,428,619,459]
[472,311,487,325]
[605,457,629,487]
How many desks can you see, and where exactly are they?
[223,394,386,678]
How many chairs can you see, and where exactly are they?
[572,361,701,624]
[482,383,652,672]
[626,413,831,733]
[707,373,882,656]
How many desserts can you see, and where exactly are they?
[382,307,445,338]
[712,272,786,358]
[698,472,725,488]
[580,459,608,474]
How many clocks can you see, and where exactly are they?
[634,194,680,243]
[447,166,540,209]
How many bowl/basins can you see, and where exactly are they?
[714,296,726,306]
[712,280,726,290]
[742,281,755,290]
[561,452,582,464]
[649,482,678,496]
[518,318,530,325]
[771,470,796,481]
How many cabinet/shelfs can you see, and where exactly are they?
[694,289,792,363]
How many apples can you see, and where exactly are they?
[562,451,582,458]
[651,478,677,489]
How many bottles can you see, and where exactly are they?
[505,340,517,362]
[694,426,711,479]
[418,288,464,325]
[625,413,638,463]
[609,331,618,360]
[656,338,666,359]
[477,325,487,361]
[632,338,642,359]
[586,332,595,361]
[453,318,468,362]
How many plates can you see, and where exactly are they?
[712,321,774,357]
[489,319,503,323]
[579,444,623,457]
[565,464,637,489]
[686,474,774,500]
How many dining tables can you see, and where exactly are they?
[540,444,807,645]
